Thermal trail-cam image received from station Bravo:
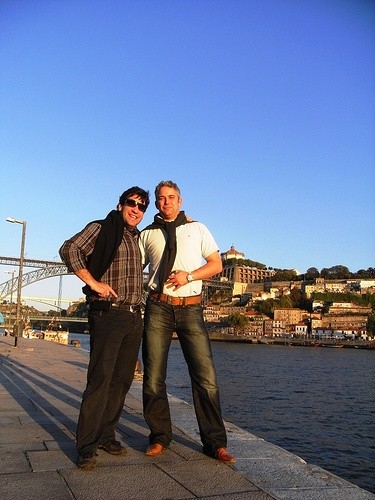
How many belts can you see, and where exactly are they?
[111,303,140,314]
[148,289,201,306]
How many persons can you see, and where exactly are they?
[131,180,236,462]
[58,185,150,470]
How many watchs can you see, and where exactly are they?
[186,272,193,283]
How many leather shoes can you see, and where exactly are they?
[145,443,165,456]
[213,448,236,463]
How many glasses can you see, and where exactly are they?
[124,198,146,213]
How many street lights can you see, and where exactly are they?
[5,216,28,347]
[5,271,14,337]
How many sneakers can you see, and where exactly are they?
[77,446,97,468]
[98,438,128,455]
